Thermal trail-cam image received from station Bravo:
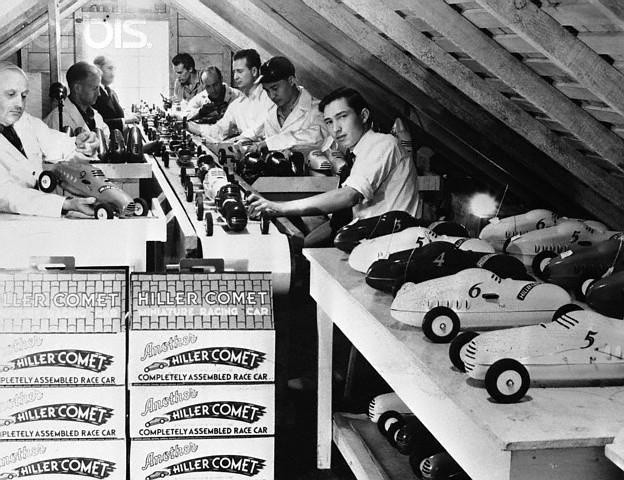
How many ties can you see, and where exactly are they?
[332,153,356,229]
[105,87,111,98]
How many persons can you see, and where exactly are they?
[185,48,273,152]
[177,66,247,124]
[229,54,331,158]
[159,50,206,107]
[40,59,164,156]
[0,60,103,220]
[93,56,143,144]
[243,85,432,392]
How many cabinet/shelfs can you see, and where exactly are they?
[300,245,624,480]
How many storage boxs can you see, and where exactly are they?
[1,264,128,332]
[130,435,277,479]
[129,383,277,437]
[1,334,129,386]
[2,386,128,440]
[128,270,277,330]
[2,440,129,479]
[127,329,275,385]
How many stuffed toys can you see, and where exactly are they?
[38,98,335,239]
[332,206,624,479]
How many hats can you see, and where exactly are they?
[253,56,295,85]
